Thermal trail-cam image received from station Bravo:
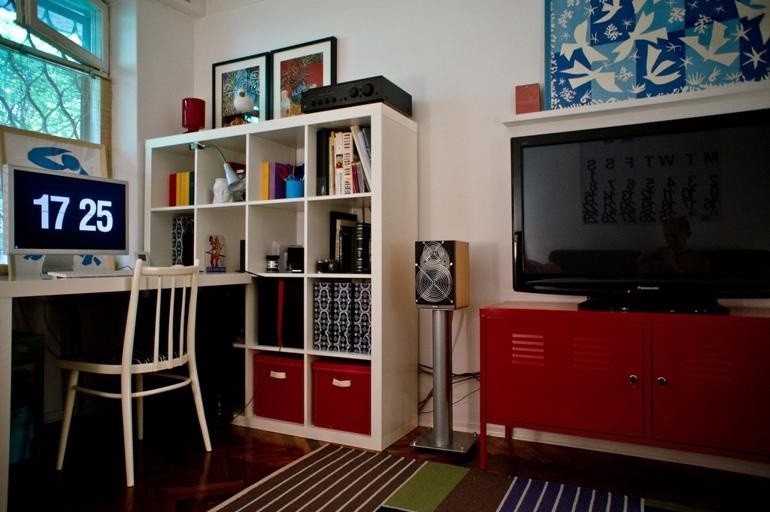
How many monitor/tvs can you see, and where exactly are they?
[509,108,770,316]
[3,163,130,280]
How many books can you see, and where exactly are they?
[261,160,270,201]
[270,161,295,200]
[315,125,372,197]
[329,210,371,274]
[168,170,195,267]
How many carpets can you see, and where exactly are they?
[207,442,703,512]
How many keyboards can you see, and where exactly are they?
[46,270,134,278]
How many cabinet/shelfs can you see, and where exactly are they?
[146,102,419,452]
[479,301,770,471]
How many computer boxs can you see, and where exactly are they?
[10,328,45,466]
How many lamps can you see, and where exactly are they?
[189,144,244,192]
[182,98,205,133]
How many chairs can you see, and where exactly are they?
[52,254,213,486]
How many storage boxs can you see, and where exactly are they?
[253,353,304,425]
[311,360,371,436]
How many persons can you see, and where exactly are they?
[636,215,716,282]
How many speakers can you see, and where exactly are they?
[414,239,471,310]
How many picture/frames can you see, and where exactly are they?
[269,36,337,120]
[0,124,117,276]
[212,51,270,129]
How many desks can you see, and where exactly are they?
[0,273,253,512]
